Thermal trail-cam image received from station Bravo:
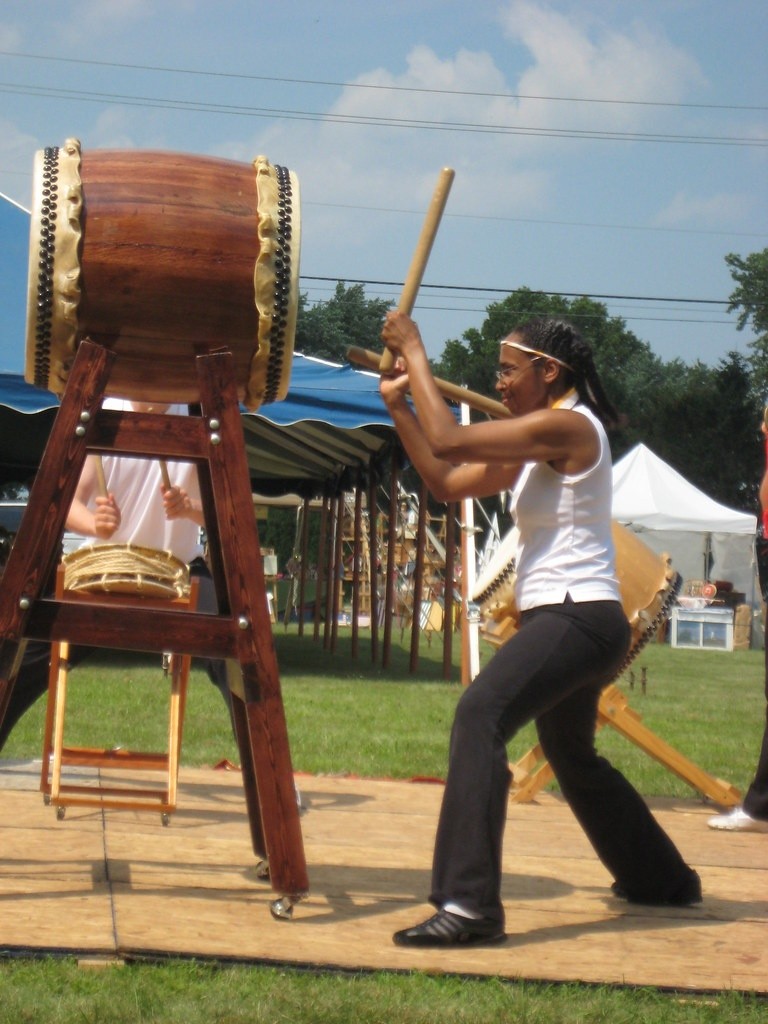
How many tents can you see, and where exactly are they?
[0,190,458,675]
[611,441,762,605]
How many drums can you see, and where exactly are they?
[23,138,303,414]
[473,506,684,685]
[63,543,188,600]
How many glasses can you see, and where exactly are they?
[495,357,541,383]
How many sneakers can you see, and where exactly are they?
[708,808,768,831]
[610,870,704,906]
[393,909,508,948]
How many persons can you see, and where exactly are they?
[0,398,307,809]
[708,402,768,830]
[379,313,702,949]
